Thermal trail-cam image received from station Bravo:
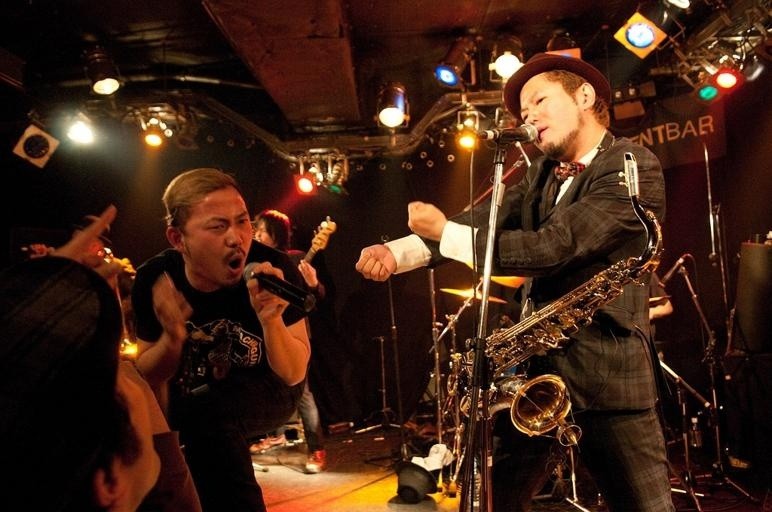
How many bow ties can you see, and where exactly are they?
[554,162,586,182]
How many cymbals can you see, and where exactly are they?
[438,288,507,305]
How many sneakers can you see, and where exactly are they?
[249,435,287,453]
[306,452,327,474]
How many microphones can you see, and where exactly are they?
[660,252,695,283]
[476,123,538,143]
[242,262,317,314]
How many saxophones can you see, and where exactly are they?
[438,151,662,436]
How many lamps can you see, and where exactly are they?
[376,28,582,150]
[139,113,163,148]
[294,152,324,197]
[713,66,741,90]
[85,45,121,96]
[741,58,765,82]
[11,115,61,168]
[613,7,668,59]
[174,109,203,152]
[698,82,717,100]
[324,153,349,197]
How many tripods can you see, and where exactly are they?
[351,336,400,434]
[659,362,713,510]
[670,272,760,508]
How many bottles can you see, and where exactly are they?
[327,421,354,434]
[688,417,703,449]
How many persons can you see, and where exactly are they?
[0,203,202,512]
[30,215,136,317]
[649,271,673,338]
[356,53,676,512]
[249,210,329,474]
[131,167,311,511]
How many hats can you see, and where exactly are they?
[395,461,438,503]
[503,53,612,126]
